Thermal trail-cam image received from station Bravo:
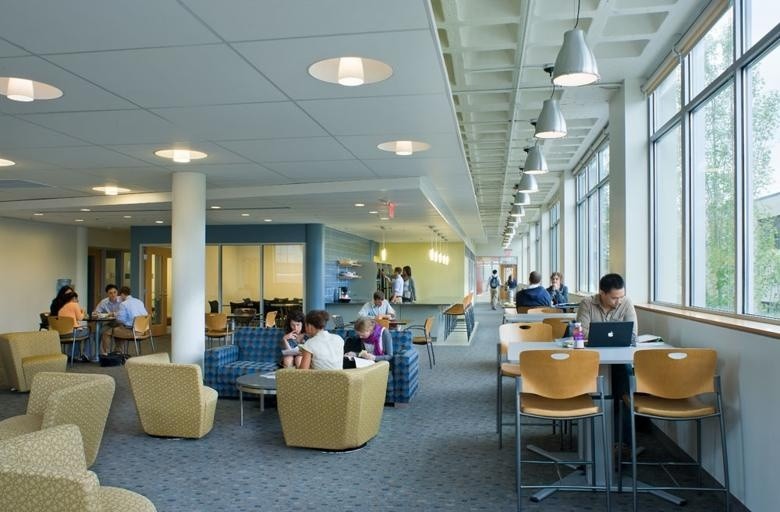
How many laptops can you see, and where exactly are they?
[584,322,634,347]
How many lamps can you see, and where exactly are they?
[498,1,602,252]
[306,54,397,88]
[379,226,388,261]
[425,225,453,268]
[377,138,432,159]
[152,147,209,166]
[91,185,133,197]
[0,74,65,106]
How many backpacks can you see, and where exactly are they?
[490,275,498,289]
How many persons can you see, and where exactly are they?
[280,311,310,368]
[574,272,646,468]
[99,286,148,357]
[50,286,73,316]
[51,292,93,362]
[546,272,568,304]
[516,271,552,313]
[401,266,416,303]
[91,284,122,334]
[507,275,516,303]
[381,267,404,303]
[344,318,393,363]
[358,291,396,322]
[297,309,345,369]
[485,270,504,310]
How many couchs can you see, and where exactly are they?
[202,322,422,408]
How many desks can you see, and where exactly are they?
[508,341,685,504]
[232,368,280,428]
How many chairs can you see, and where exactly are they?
[0,423,160,512]
[0,369,118,471]
[515,350,610,511]
[617,349,730,512]
[498,305,580,448]
[1,328,70,394]
[330,291,478,369]
[123,350,217,438]
[273,360,393,453]
[33,293,303,366]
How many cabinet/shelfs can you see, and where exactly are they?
[335,261,363,279]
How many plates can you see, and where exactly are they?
[83,312,116,319]
[503,300,516,305]
[365,313,397,321]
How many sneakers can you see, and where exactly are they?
[630,442,645,460]
[81,352,91,363]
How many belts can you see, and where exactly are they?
[391,296,401,298]
[123,324,133,330]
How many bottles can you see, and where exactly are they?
[573,321,584,349]
[506,289,514,303]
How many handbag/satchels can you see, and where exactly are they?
[98,350,132,367]
